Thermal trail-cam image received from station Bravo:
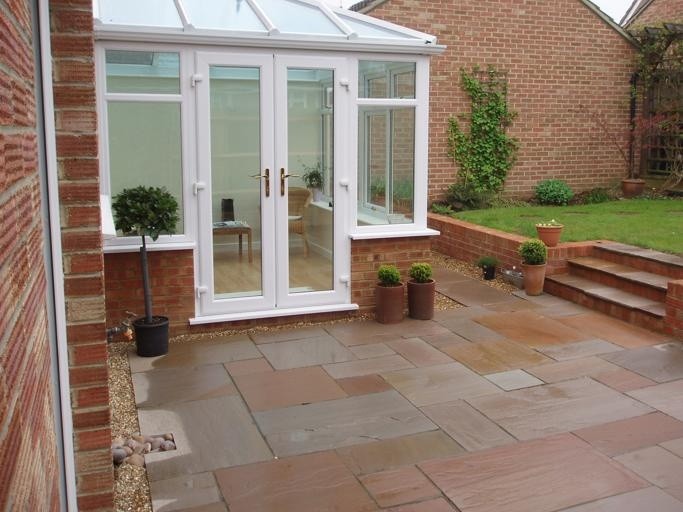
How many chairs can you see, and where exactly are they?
[288,186,313,259]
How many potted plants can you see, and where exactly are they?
[477,257,498,281]
[519,237,549,296]
[109,182,181,358]
[407,263,436,321]
[299,161,327,203]
[621,112,683,199]
[374,263,406,326]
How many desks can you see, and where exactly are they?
[212,221,252,263]
